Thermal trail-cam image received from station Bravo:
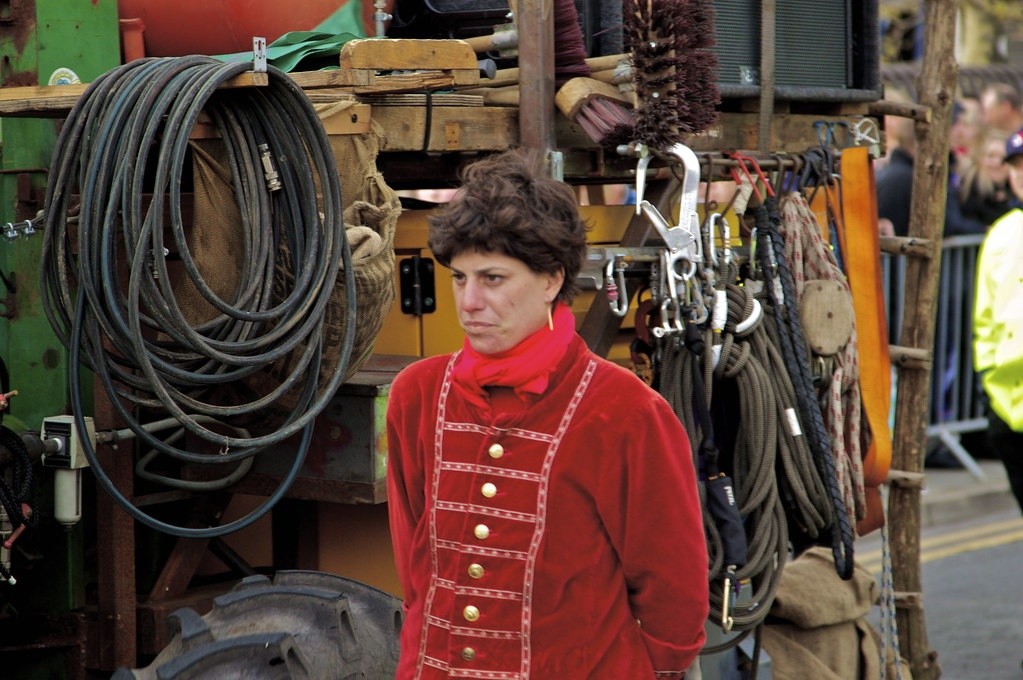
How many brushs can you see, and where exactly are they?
[554,103,598,153]
[462,0,594,79]
[621,0,723,149]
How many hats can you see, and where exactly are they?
[1002,127,1023,164]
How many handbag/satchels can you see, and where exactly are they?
[267,172,402,409]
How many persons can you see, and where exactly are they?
[878,87,1023,241]
[880,11,916,61]
[383,151,710,680]
[971,129,1023,510]
